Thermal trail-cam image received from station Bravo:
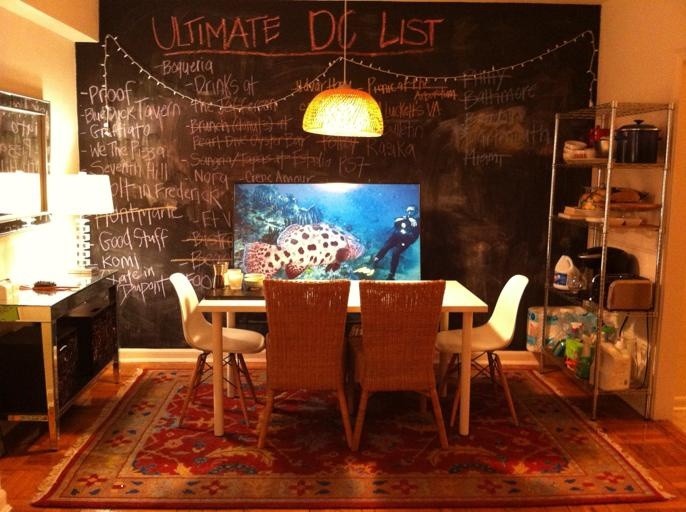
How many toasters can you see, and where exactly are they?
[591,271,652,310]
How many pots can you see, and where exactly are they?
[614,119,662,163]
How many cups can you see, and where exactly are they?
[567,274,585,295]
[212,261,264,291]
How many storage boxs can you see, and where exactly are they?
[526,305,587,353]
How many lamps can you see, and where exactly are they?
[48,171,114,274]
[302,0,385,138]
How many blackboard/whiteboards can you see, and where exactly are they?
[76,1,601,350]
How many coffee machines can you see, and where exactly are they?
[578,246,627,301]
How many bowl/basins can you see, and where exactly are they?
[564,128,616,164]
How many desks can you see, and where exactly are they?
[1,266,119,452]
[195,280,489,438]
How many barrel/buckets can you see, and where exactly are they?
[553,255,580,290]
[589,338,632,391]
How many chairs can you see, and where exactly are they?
[347,279,450,451]
[433,274,531,428]
[257,279,354,450]
[168,272,266,428]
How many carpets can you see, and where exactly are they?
[29,365,679,510]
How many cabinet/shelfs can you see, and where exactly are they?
[537,100,675,420]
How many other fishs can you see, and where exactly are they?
[243,222,365,292]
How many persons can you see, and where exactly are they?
[366,204,420,280]
